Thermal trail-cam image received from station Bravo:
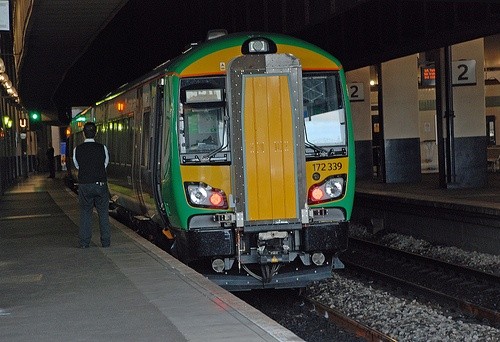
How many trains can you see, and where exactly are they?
[64,27,356,290]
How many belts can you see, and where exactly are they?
[96,181,106,186]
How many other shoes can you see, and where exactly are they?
[80,245,86,248]
[102,245,109,248]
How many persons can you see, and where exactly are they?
[46,143,55,178]
[73,122,111,248]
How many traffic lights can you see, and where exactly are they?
[30,109,40,128]
